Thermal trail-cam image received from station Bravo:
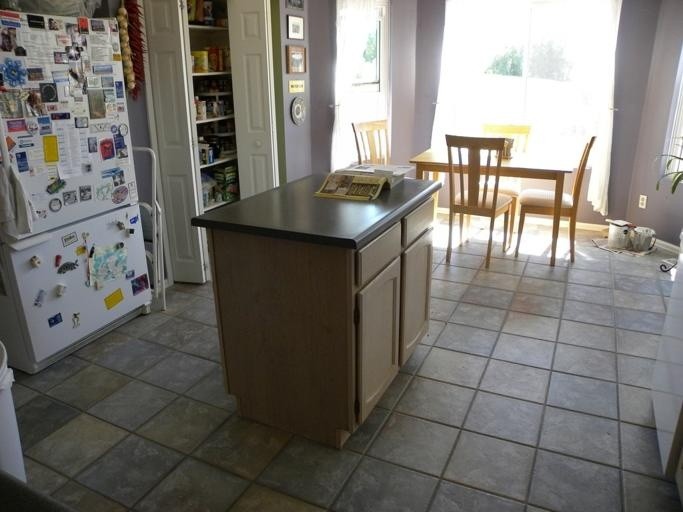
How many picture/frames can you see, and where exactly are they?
[286,13,304,41]
[285,44,306,75]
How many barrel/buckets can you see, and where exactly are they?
[630,226,656,253]
[607,223,630,250]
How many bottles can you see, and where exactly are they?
[194,96,202,121]
[195,80,220,93]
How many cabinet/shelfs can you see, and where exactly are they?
[191,174,441,451]
[185,21,241,212]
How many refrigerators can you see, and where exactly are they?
[0,10,155,375]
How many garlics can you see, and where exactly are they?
[117,7,135,89]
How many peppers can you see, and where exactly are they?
[125,0,145,102]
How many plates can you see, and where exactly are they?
[291,97,306,126]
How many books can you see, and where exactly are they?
[334,162,417,176]
[312,170,405,204]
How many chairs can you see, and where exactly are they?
[443,134,510,270]
[466,121,531,247]
[350,119,415,180]
[513,132,596,263]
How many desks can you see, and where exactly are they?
[410,144,572,267]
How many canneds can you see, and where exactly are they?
[202,147,214,164]
